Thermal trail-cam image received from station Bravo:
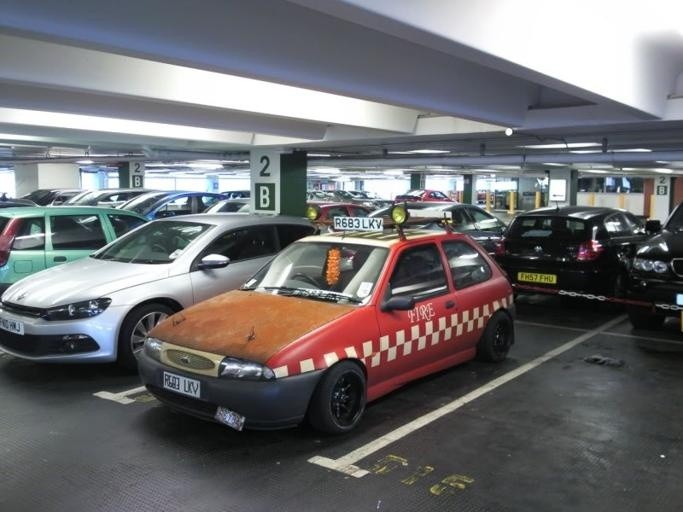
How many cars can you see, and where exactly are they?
[634,195,683,331]
[2,188,159,208]
[201,199,262,216]
[307,201,365,225]
[397,189,453,205]
[149,192,227,217]
[304,189,370,203]
[498,205,654,302]
[223,189,251,200]
[138,210,517,432]
[1,216,322,365]
[1,206,144,284]
[371,201,507,252]
